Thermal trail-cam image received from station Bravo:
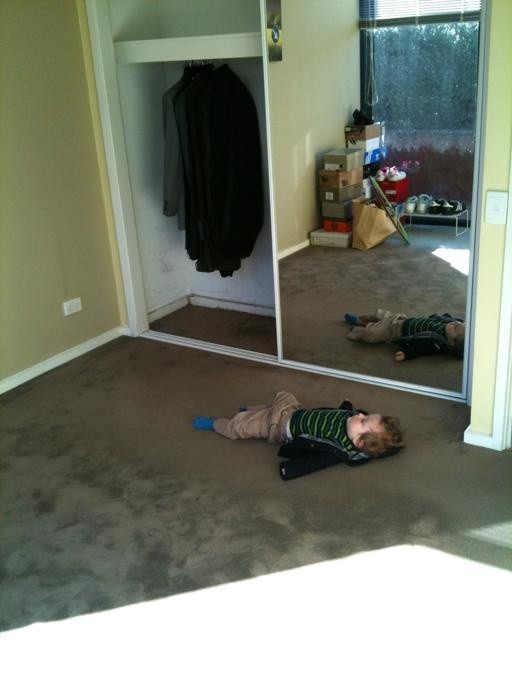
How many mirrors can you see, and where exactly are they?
[261,0,493,406]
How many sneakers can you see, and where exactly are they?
[443,198,466,215]
[429,196,443,214]
[374,166,390,182]
[417,193,433,214]
[405,195,418,214]
[388,165,407,182]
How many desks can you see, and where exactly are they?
[395,203,468,236]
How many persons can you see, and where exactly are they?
[192,391,405,481]
[344,309,465,362]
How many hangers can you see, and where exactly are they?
[185,60,227,68]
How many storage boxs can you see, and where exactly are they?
[310,121,385,247]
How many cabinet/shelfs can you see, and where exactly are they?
[84,0,281,363]
